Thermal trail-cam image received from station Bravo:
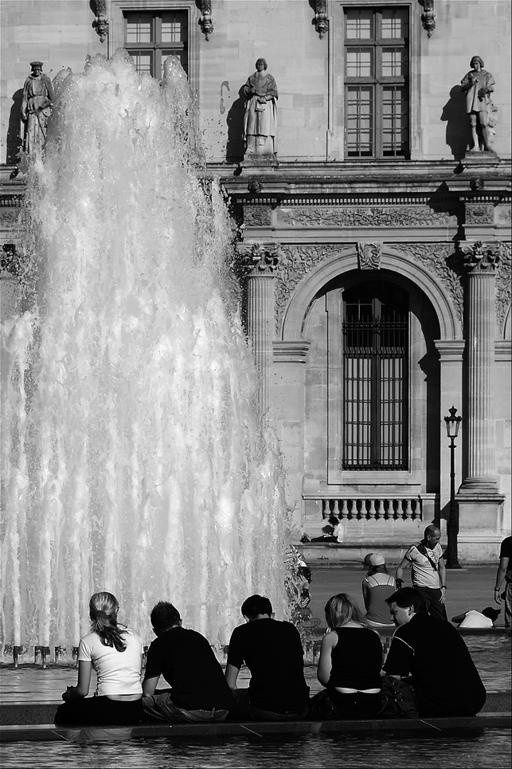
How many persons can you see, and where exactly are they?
[238,58,279,154]
[451,605,502,630]
[359,552,397,627]
[311,515,345,542]
[20,60,55,149]
[311,592,384,720]
[52,590,144,727]
[141,600,232,724]
[225,594,311,721]
[396,525,449,622]
[493,535,512,631]
[382,586,488,716]
[460,55,496,151]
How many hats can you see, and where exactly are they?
[363,551,386,566]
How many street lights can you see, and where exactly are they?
[441,405,461,570]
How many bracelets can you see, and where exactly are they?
[495,586,500,592]
[441,586,447,589]
